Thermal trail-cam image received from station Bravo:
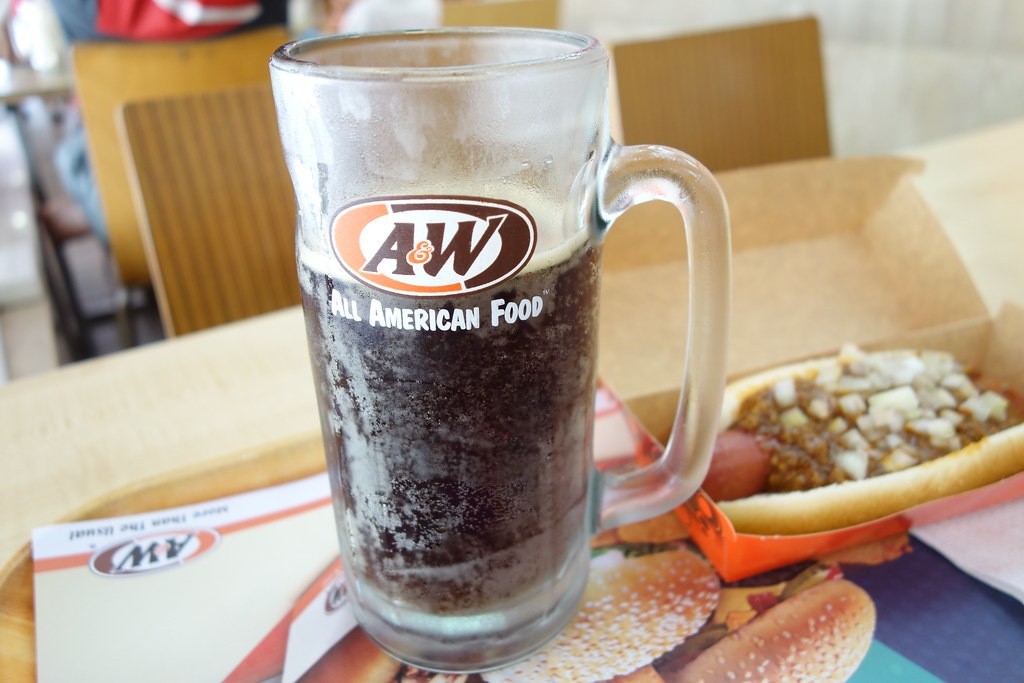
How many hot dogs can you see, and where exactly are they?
[695,342,1024,536]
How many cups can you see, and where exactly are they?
[267,24,738,674]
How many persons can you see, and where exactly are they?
[10,0,443,315]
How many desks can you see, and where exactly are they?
[0,121,1024,683]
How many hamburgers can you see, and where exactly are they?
[283,507,875,683]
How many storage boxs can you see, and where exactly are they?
[592,148,1024,585]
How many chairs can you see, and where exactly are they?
[69,0,822,343]
